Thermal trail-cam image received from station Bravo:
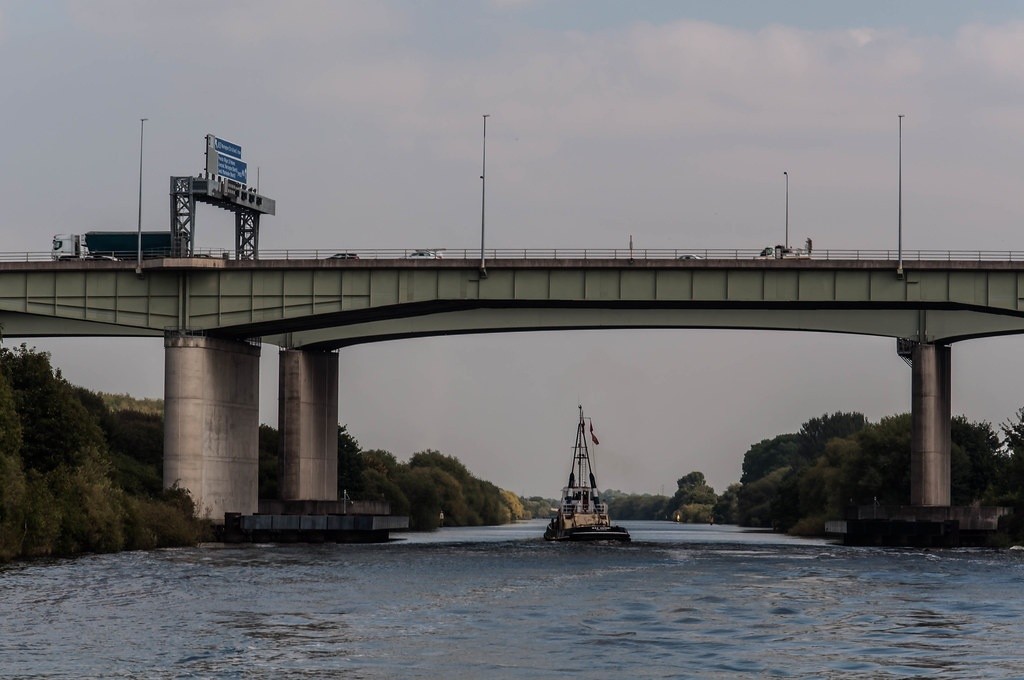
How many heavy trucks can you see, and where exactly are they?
[50,231,189,259]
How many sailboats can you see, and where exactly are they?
[543,403,632,545]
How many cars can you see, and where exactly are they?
[678,255,704,259]
[400,252,442,259]
[326,252,359,259]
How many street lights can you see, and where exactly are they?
[784,172,789,248]
[137,118,149,260]
[481,114,491,267]
[897,114,906,269]
